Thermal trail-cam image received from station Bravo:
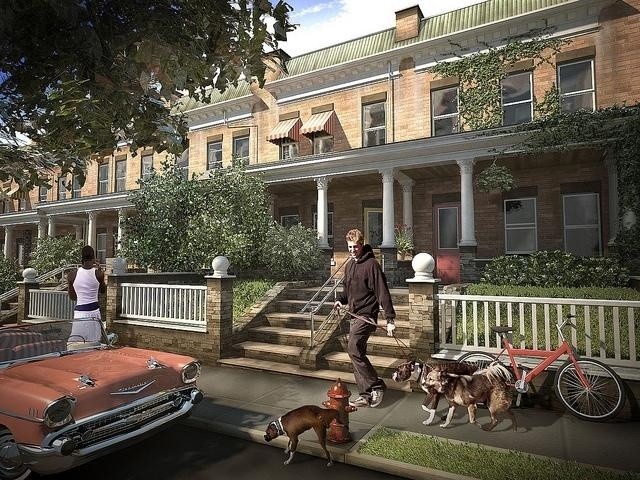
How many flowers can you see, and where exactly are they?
[394,224,413,252]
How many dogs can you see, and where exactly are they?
[420,364,518,433]
[263,403,343,468]
[392,358,479,426]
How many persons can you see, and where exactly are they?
[332,227,398,408]
[66,244,109,350]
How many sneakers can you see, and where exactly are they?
[371,383,388,408]
[348,396,370,409]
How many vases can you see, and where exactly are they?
[397,249,406,260]
